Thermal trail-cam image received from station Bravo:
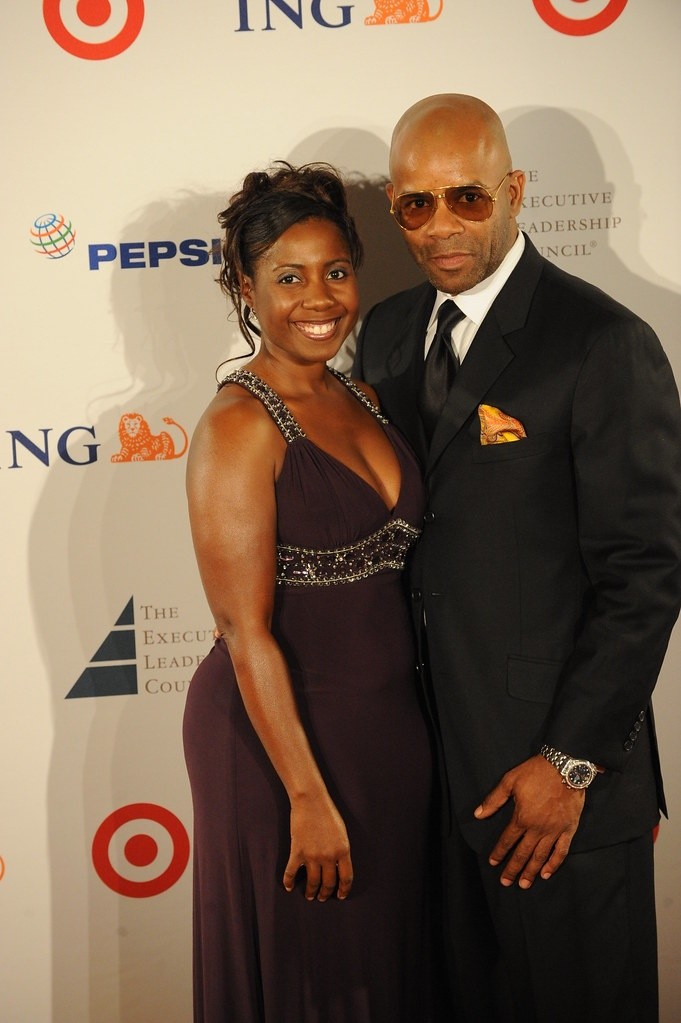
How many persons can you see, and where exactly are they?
[183,162,448,1023]
[360,94,681,1023]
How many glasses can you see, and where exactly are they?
[388,172,513,231]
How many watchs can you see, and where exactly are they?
[541,745,597,790]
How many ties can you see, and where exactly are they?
[417,299,467,454]
